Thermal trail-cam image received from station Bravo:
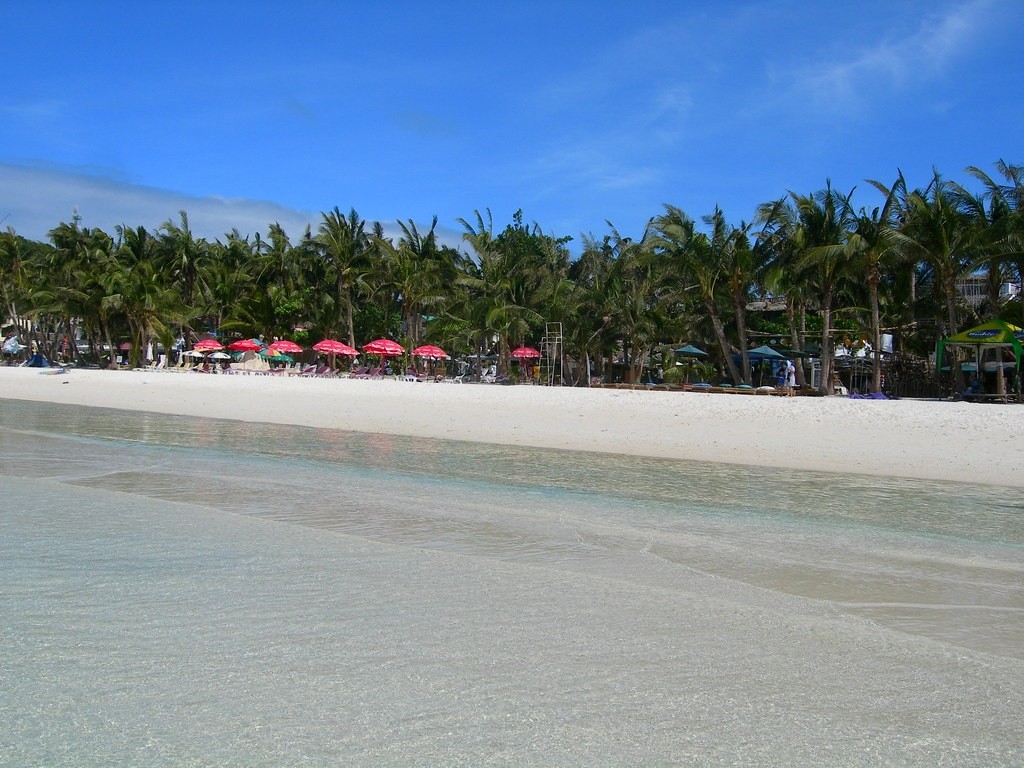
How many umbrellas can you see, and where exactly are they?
[272,355,293,361]
[410,345,448,371]
[746,346,784,387]
[248,338,264,345]
[227,340,262,352]
[363,339,405,375]
[193,339,222,349]
[182,351,205,363]
[268,341,303,352]
[512,347,542,371]
[312,340,360,367]
[207,352,231,362]
[675,345,708,383]
[260,349,280,357]
[781,349,809,358]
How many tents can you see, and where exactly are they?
[937,319,1024,402]
[23,354,49,367]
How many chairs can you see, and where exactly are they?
[591,382,803,396]
[396,373,466,384]
[133,360,380,380]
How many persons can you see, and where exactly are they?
[657,365,663,383]
[395,363,399,371]
[775,361,795,396]
[351,366,359,373]
[408,364,420,377]
[491,363,496,374]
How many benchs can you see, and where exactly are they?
[962,393,1016,404]
